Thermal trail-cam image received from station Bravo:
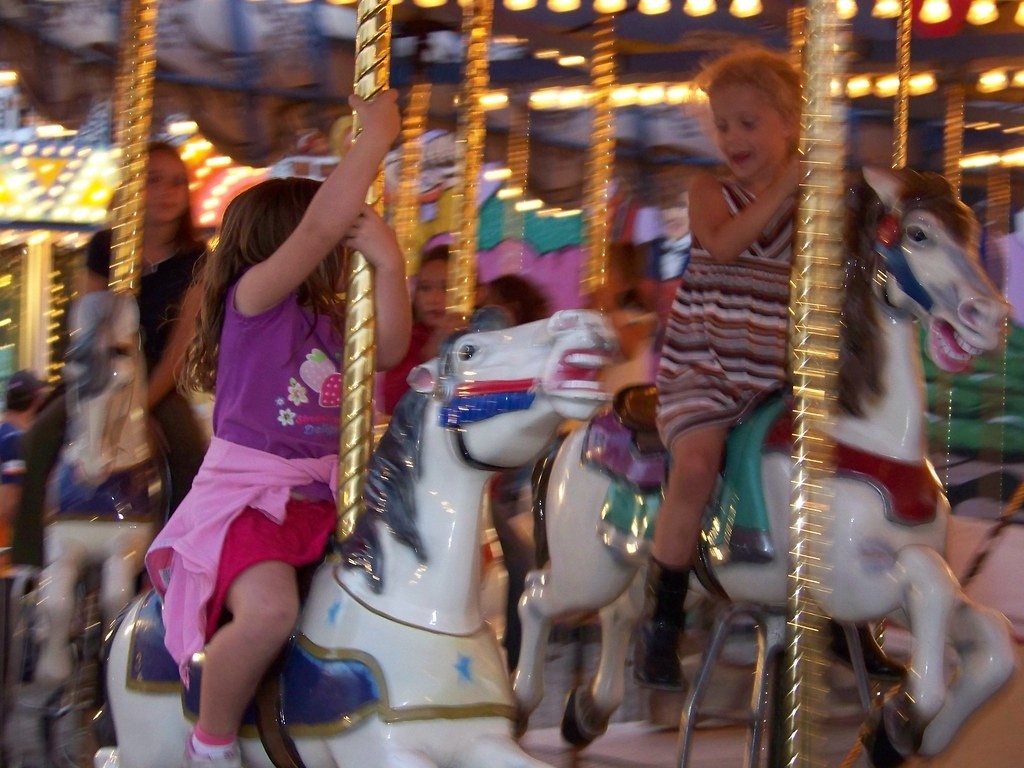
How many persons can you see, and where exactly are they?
[841,154,885,251]
[634,47,907,693]
[145,88,412,768]
[0,142,547,689]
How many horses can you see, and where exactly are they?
[4,565,88,767]
[39,293,174,676]
[104,303,612,768]
[510,166,1014,768]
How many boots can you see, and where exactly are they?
[824,618,906,682]
[632,552,686,693]
